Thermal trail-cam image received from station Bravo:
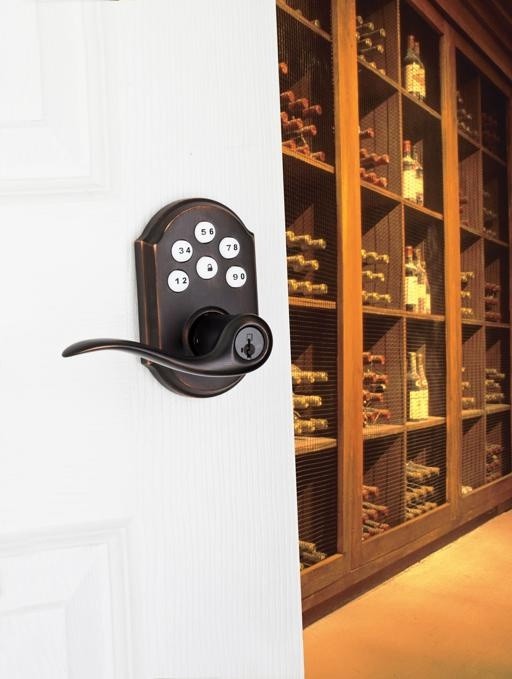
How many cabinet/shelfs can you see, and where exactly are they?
[275,1,510,627]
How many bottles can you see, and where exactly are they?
[278,7,505,582]
